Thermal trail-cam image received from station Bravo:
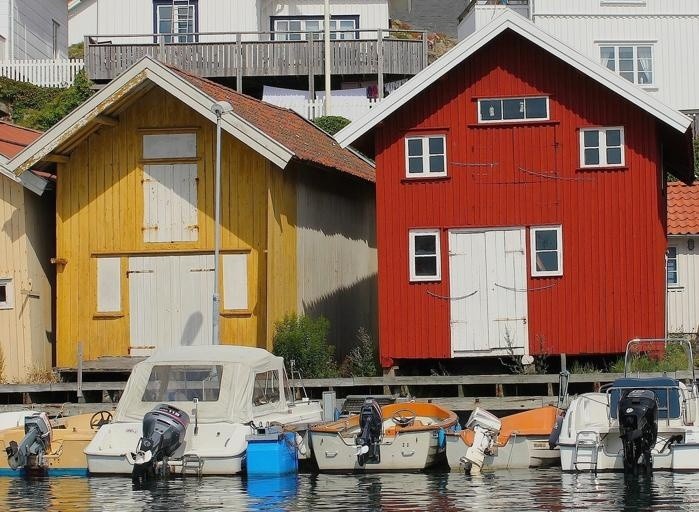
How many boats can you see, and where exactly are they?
[554,337,698,474]
[79,344,326,480]
[305,399,459,475]
[442,403,567,473]
[0,411,40,431]
[0,408,115,478]
